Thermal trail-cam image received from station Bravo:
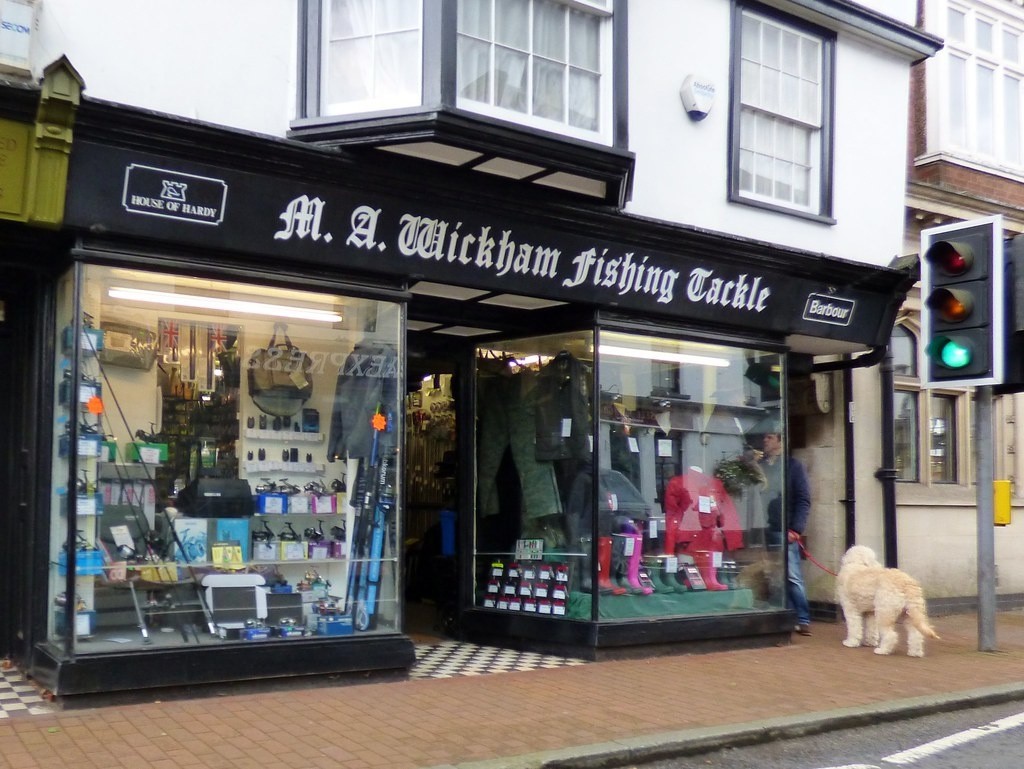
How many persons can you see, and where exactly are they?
[756,429,813,636]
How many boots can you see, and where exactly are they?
[622,532,653,594]
[717,566,738,590]
[577,536,613,595]
[657,554,688,592]
[644,554,675,594]
[610,532,642,594]
[695,549,728,590]
[713,567,728,590]
[719,560,746,590]
[590,536,627,595]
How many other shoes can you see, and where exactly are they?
[798,623,813,636]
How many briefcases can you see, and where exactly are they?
[179,476,255,519]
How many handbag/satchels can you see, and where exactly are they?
[98,321,158,370]
[247,323,313,418]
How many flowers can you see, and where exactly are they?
[712,458,763,498]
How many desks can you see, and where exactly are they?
[194,571,270,631]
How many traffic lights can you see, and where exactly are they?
[919,214,1005,389]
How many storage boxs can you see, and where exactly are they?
[60,494,103,515]
[258,492,346,514]
[60,380,102,403]
[317,615,353,636]
[155,513,262,564]
[300,583,327,603]
[125,442,168,462]
[239,627,305,640]
[58,432,116,463]
[141,562,178,582]
[59,551,104,574]
[56,611,97,636]
[61,326,104,358]
[253,538,346,561]
[302,408,320,432]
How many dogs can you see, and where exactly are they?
[731,554,781,615]
[836,546,941,658]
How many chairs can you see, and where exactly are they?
[96,504,215,645]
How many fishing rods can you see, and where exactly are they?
[82,323,224,642]
[84,377,189,644]
[82,340,203,646]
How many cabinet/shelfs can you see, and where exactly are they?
[245,429,326,471]
[162,394,239,477]
[250,513,372,565]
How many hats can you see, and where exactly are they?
[434,450,459,484]
